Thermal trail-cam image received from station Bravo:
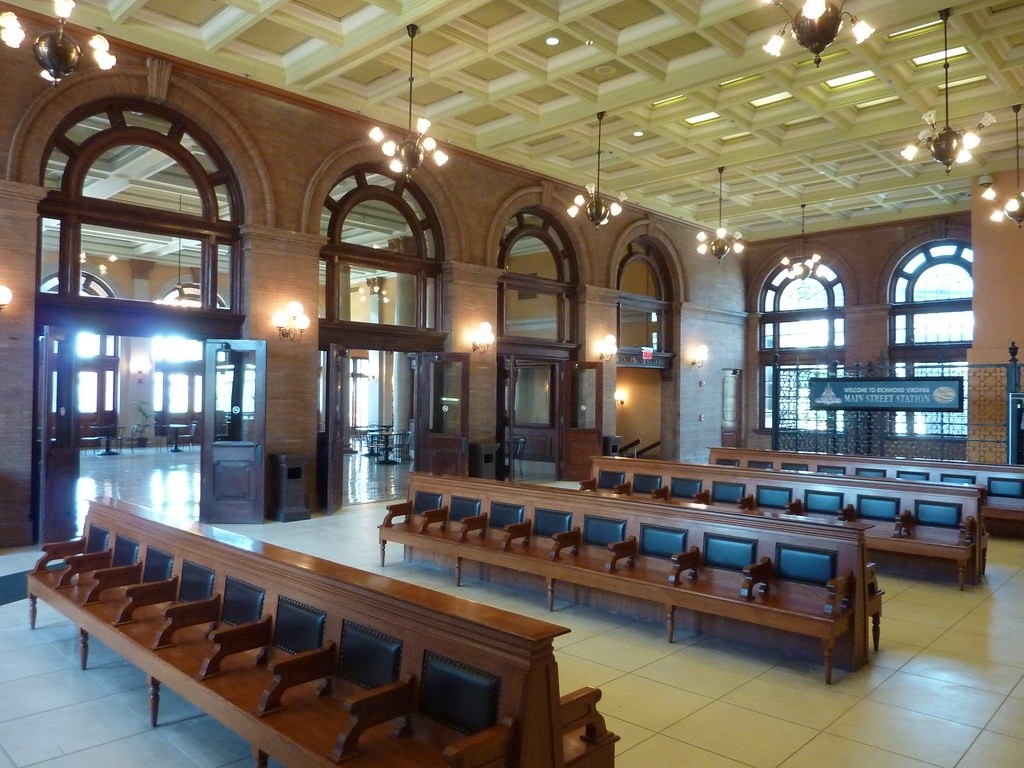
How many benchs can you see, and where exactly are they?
[0,447,1024,768]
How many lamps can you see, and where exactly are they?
[0,284,11,309]
[272,298,310,343]
[593,334,618,363]
[782,205,827,280]
[466,321,497,354]
[980,104,1024,231]
[615,388,628,405]
[176,195,185,289]
[566,112,627,230]
[692,344,708,368]
[760,0,876,66]
[368,24,448,174]
[0,0,117,84]
[899,8,998,173]
[696,166,744,262]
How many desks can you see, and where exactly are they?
[367,432,411,464]
[355,427,388,456]
[90,425,125,456]
[163,424,191,452]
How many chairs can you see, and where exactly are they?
[83,421,199,456]
[505,438,526,479]
[367,425,410,463]
